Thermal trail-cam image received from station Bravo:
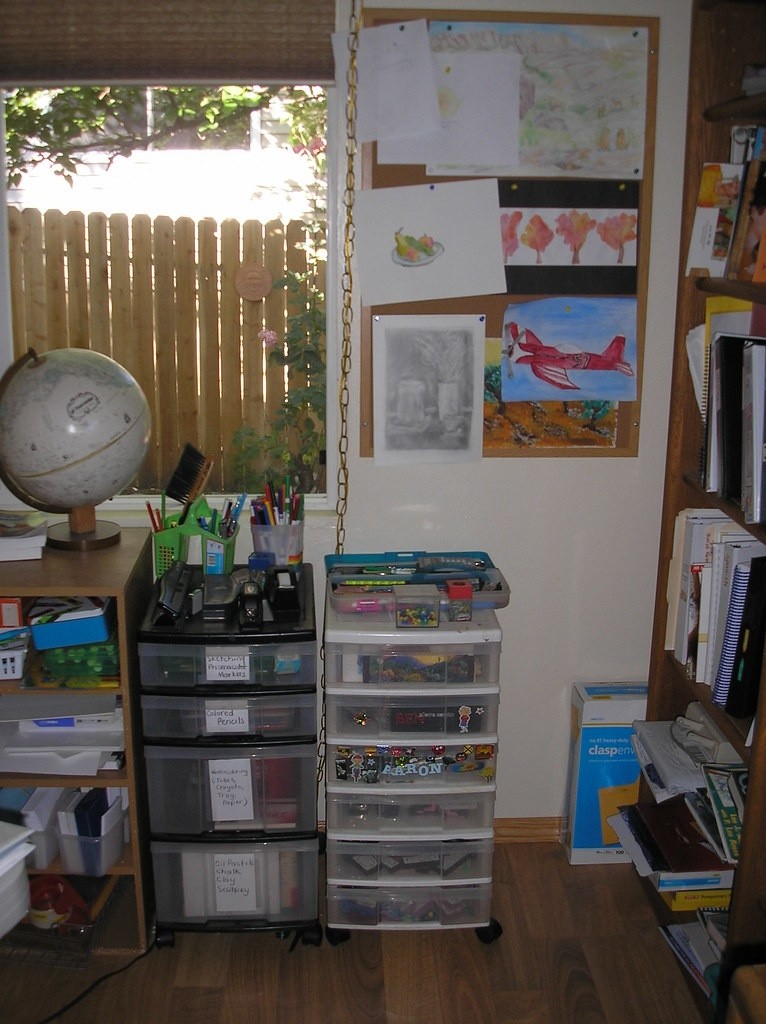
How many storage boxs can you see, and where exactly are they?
[324,549,511,629]
[0,596,130,873]
[609,805,734,912]
[251,516,306,563]
[557,680,648,865]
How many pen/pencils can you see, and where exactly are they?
[248,475,306,563]
[339,577,412,593]
[195,494,248,542]
[144,493,194,533]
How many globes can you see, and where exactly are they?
[0,345,154,555]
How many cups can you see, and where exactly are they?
[250,524,303,573]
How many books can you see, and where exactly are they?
[664,124,766,748]
[0,691,125,877]
[0,509,49,562]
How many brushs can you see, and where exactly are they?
[162,443,216,527]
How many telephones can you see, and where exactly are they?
[235,579,265,636]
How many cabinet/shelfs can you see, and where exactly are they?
[322,586,503,931]
[0,528,151,957]
[634,1,766,1024]
[133,563,324,936]
